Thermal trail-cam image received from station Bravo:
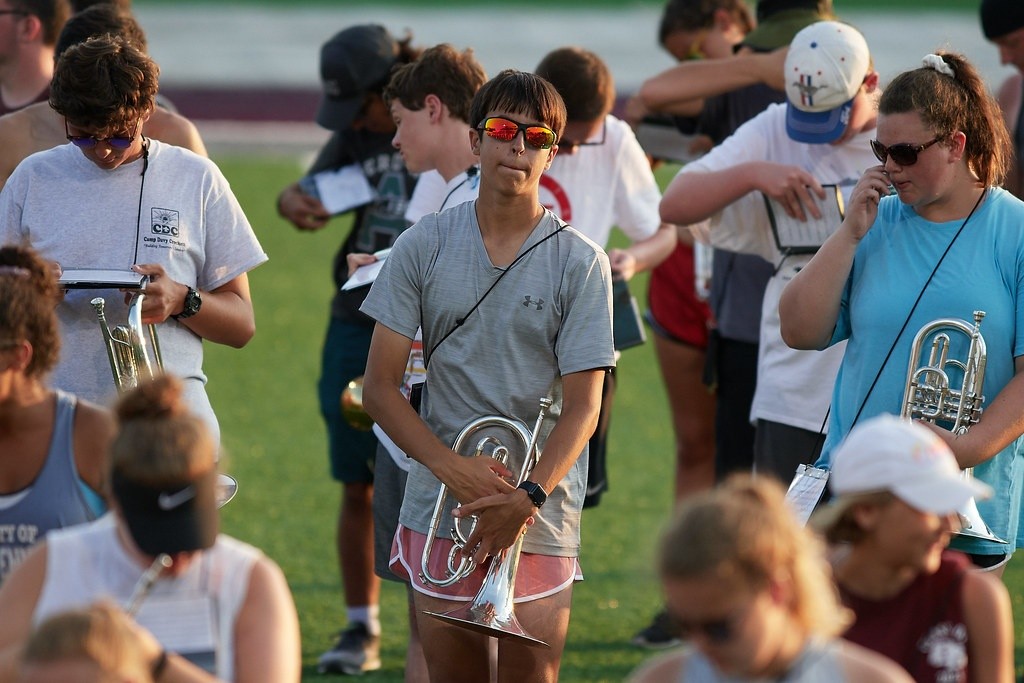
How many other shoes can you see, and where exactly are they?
[631,609,681,649]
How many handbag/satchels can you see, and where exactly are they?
[406,382,424,458]
[784,464,830,528]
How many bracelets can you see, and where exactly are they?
[150,649,169,682]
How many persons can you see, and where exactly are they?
[275,2,1024,683]
[0,0,302,683]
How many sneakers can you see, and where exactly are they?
[315,620,382,675]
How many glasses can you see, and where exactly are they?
[686,6,717,60]
[475,117,556,148]
[559,116,606,150]
[870,132,952,166]
[670,586,761,645]
[65,109,140,150]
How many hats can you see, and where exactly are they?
[106,446,219,554]
[832,414,995,511]
[782,21,870,142]
[317,24,400,130]
[744,8,824,49]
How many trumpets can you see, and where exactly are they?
[898,308,1011,547]
[418,396,555,652]
[90,276,238,512]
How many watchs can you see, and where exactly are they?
[518,480,547,510]
[170,285,203,321]
